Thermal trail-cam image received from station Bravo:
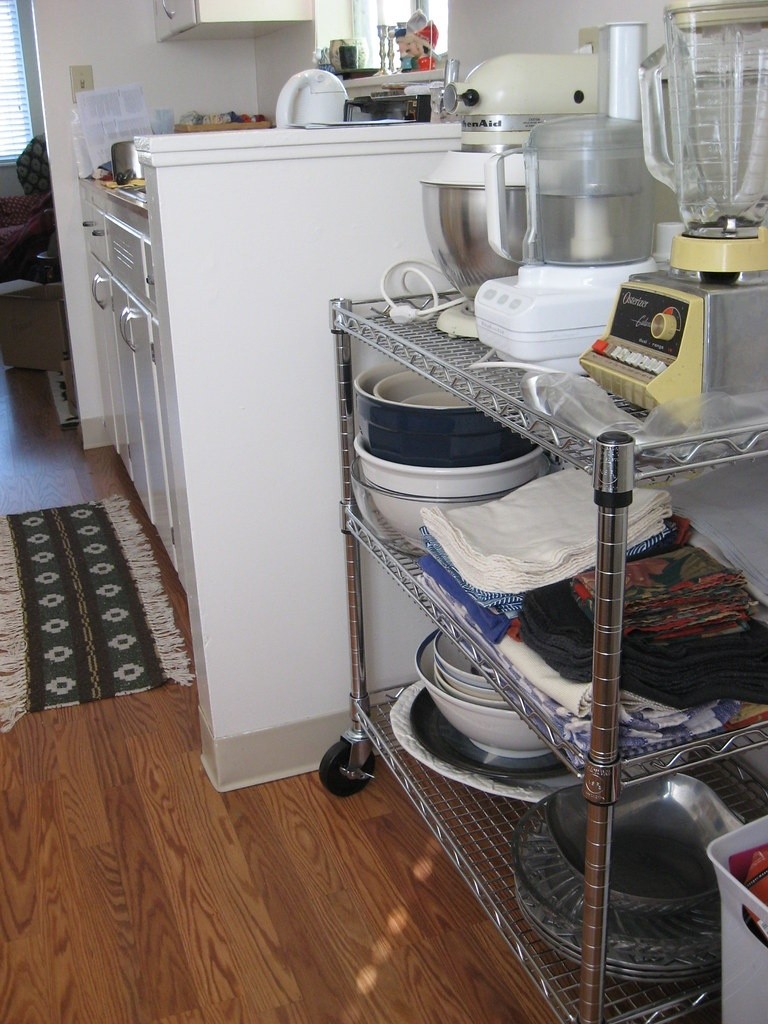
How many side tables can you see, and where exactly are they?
[37,252,60,284]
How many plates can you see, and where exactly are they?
[511,774,750,981]
[389,680,582,802]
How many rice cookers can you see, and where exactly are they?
[421,52,598,336]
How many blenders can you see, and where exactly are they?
[475,113,657,377]
[581,2,766,424]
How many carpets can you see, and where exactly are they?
[0,495,195,734]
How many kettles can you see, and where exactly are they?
[275,68,347,129]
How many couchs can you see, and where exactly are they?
[0,188,51,280]
[0,278,68,370]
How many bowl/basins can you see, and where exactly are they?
[546,774,746,911]
[414,627,573,758]
[348,362,554,548]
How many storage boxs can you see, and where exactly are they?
[706,813,767,1024]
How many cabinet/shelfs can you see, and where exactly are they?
[152,0,314,43]
[80,176,134,492]
[110,189,429,591]
[326,285,767,1022]
